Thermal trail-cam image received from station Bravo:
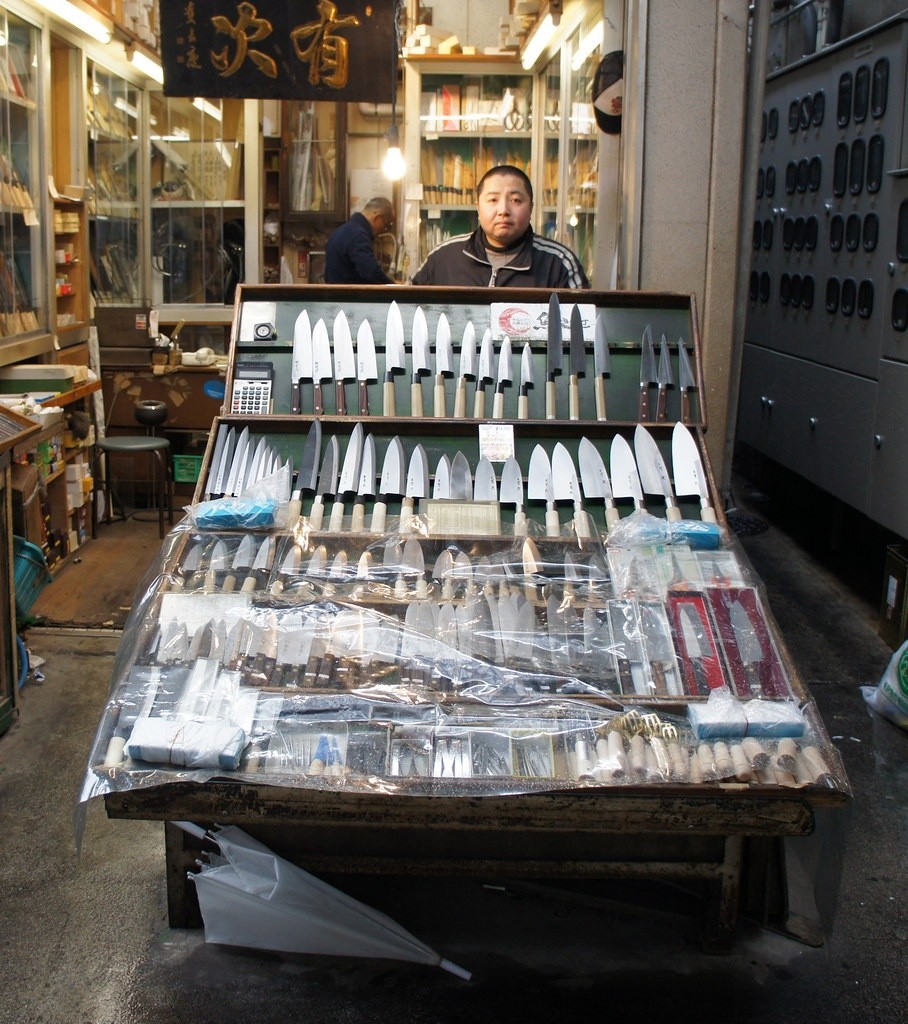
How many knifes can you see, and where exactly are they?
[1,40,209,457]
[108,291,852,790]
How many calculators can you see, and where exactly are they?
[230,360,274,415]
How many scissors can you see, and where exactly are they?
[547,99,560,132]
[504,97,525,130]
[711,559,731,586]
[666,552,698,591]
[622,556,659,600]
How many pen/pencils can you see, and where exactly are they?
[174,334,178,350]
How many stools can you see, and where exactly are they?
[98,435,182,540]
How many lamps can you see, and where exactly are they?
[520,0,563,70]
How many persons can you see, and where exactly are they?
[322,195,394,285]
[410,165,590,290]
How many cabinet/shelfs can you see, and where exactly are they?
[75,42,224,308]
[0,0,58,367]
[530,0,627,289]
[398,53,540,284]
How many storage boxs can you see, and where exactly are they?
[173,454,204,482]
[67,461,95,552]
[12,534,56,619]
[400,25,479,55]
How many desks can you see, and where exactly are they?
[11,378,103,577]
[103,593,851,947]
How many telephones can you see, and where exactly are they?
[181,346,217,366]
